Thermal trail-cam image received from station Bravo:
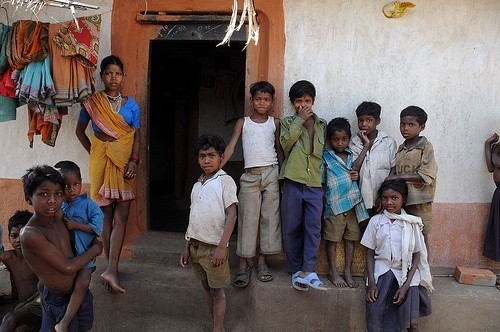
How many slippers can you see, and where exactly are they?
[233,266,250,287]
[255,263,273,282]
[297,273,327,290]
[292,271,309,291]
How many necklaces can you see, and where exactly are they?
[105,91,122,114]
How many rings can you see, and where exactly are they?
[127,171,130,174]
[134,174,137,176]
[132,174,134,177]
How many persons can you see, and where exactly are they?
[320,117,370,289]
[483,133,500,262]
[179,133,238,332]
[348,100,398,233]
[0,210,42,332]
[383,106,438,265]
[197,81,284,289]
[76,55,142,296]
[19,164,103,332]
[278,81,328,291]
[360,179,433,332]
[53,161,105,331]
